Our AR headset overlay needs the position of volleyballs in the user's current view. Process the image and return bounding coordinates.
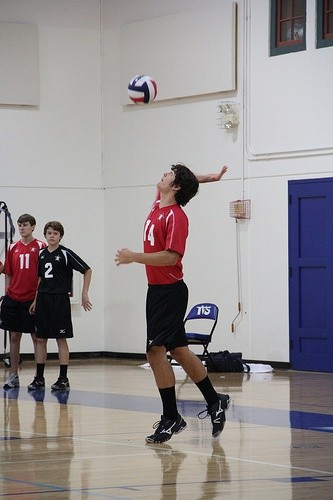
[128,74,158,105]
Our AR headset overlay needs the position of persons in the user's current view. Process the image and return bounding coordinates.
[0,261,4,274]
[27,221,92,390]
[114,163,231,442]
[0,214,47,390]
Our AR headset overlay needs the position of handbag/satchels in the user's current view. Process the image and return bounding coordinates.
[208,350,250,373]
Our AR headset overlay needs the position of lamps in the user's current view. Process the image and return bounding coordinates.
[218,102,240,128]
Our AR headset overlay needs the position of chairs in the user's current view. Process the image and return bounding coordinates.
[169,303,219,371]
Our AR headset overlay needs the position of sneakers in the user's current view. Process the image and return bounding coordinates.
[198,393,230,438]
[3,373,19,387]
[145,413,187,443]
[28,376,45,390]
[51,376,69,390]
